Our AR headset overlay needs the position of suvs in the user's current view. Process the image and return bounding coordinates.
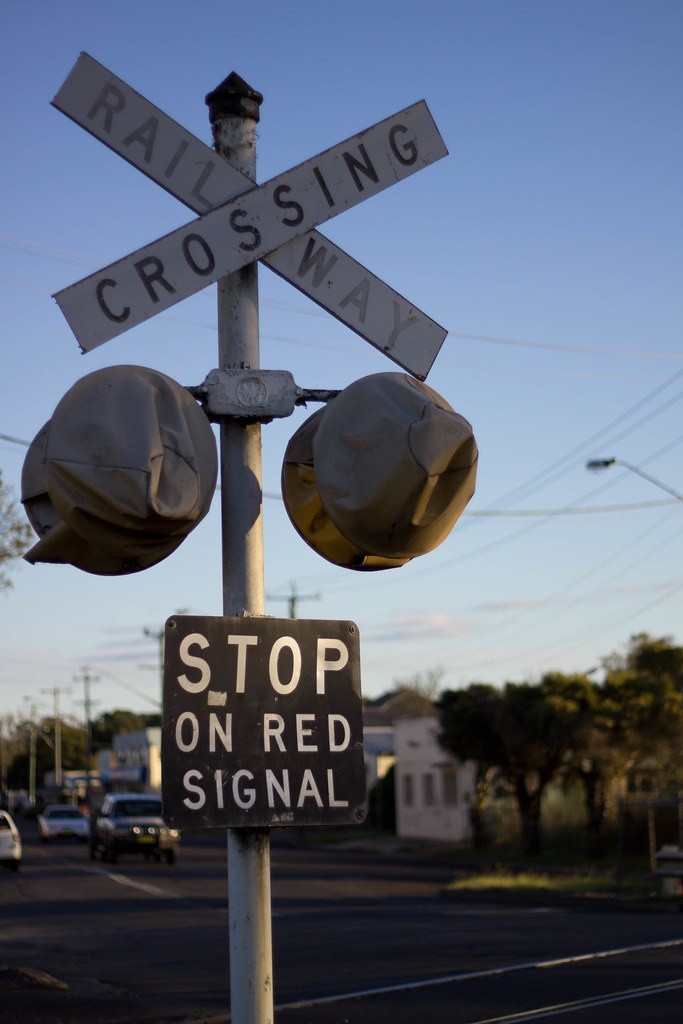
[89,791,181,866]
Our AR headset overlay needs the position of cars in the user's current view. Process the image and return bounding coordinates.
[0,810,22,872]
[37,804,89,844]
